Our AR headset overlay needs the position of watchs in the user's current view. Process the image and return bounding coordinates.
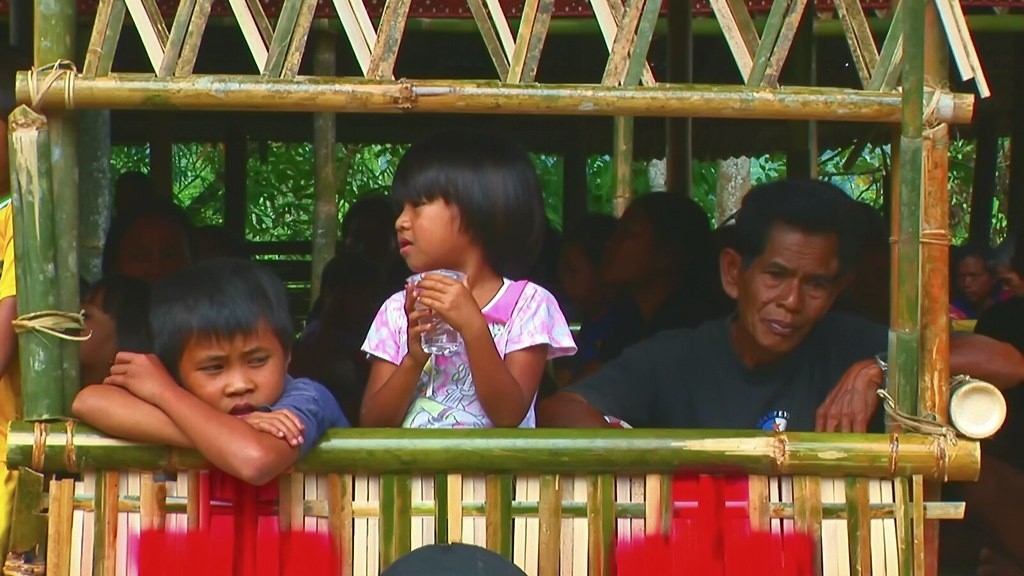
[872,350,888,392]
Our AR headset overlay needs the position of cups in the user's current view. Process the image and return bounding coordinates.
[406,269,469,355]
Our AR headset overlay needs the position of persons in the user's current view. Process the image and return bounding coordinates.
[288,130,1023,576]
[70,252,352,486]
[0,107,228,576]
